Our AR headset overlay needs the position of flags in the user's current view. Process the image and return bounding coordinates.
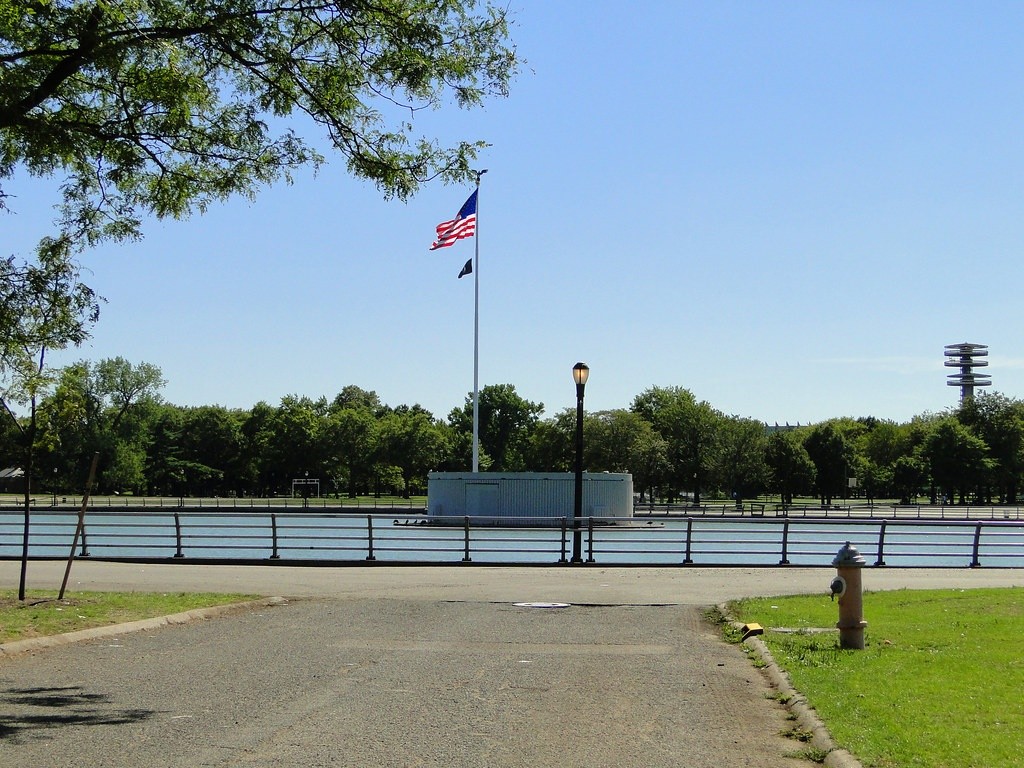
[458,258,473,278]
[458,258,472,278]
[429,187,478,251]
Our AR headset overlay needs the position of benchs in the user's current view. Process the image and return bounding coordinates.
[757,496,778,503]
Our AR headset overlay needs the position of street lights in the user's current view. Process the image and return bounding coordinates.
[571,360,590,564]
[53,468,58,505]
[180,469,184,506]
[305,471,309,508]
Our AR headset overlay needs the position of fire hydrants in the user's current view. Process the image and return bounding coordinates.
[824,542,867,649]
